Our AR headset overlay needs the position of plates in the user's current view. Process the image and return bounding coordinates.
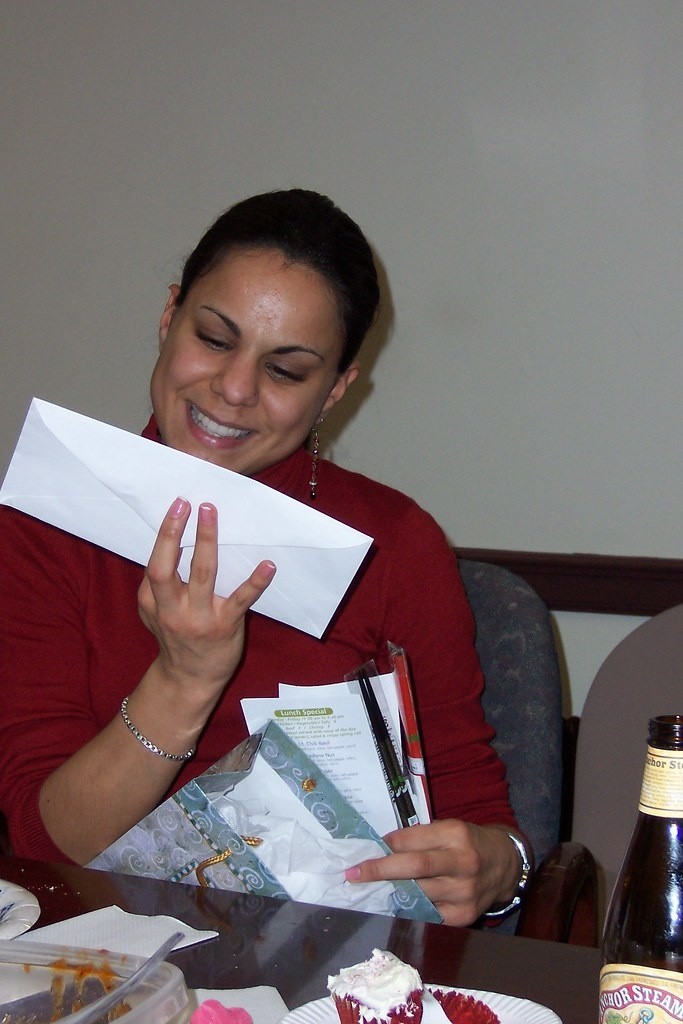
[278,983,562,1023]
[0,879,41,941]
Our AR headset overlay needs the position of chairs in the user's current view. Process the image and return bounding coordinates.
[448,560,683,951]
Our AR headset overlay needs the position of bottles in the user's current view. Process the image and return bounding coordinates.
[598,715,683,1024]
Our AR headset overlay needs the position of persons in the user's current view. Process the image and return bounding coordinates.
[0,185,532,931]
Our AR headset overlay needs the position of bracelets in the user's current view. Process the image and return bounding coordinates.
[482,827,532,919]
[118,695,198,763]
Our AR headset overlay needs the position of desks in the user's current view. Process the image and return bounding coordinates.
[0,854,683,1024]
